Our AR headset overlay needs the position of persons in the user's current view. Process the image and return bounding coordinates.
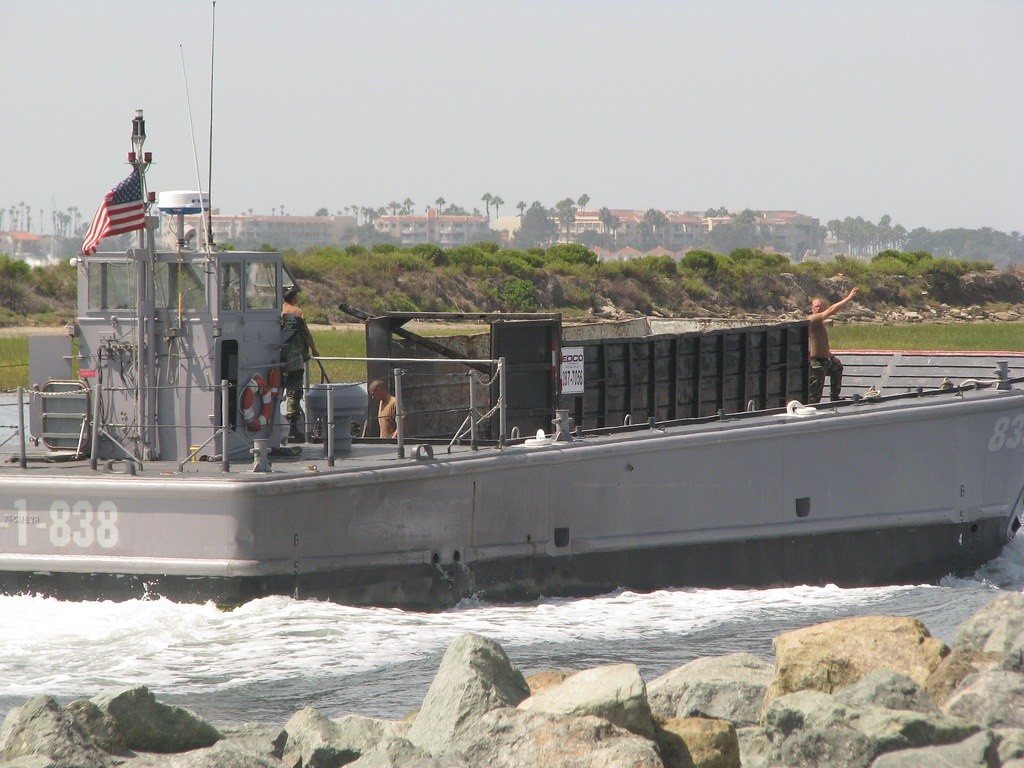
[809,287,861,403]
[370,380,407,438]
[283,288,319,437]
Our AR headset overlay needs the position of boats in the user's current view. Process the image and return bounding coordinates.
[0,0,1023,612]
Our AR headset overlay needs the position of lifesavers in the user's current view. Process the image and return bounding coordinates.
[243,377,272,430]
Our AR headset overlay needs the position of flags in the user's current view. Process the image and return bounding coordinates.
[82,170,145,256]
